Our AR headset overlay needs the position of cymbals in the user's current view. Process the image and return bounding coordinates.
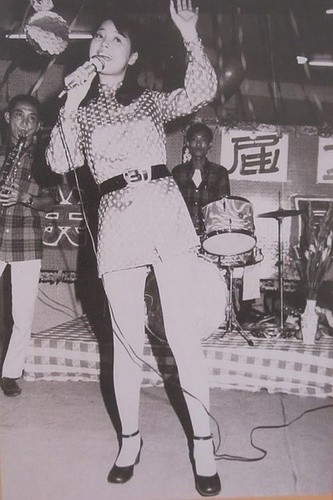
[256,209,302,218]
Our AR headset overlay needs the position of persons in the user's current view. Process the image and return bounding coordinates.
[0,94,58,398]
[170,122,264,323]
[46,0,221,496]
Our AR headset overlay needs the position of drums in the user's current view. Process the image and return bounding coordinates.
[200,196,256,256]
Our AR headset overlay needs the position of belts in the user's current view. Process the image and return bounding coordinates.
[97,163,173,195]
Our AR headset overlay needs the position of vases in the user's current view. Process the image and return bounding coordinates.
[301,300,318,344]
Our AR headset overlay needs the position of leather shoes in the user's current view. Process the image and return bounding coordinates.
[192,434,221,497]
[0,377,22,397]
[107,430,144,484]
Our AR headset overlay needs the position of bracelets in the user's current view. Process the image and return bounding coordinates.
[26,194,33,206]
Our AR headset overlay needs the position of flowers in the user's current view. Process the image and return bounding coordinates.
[293,203,333,300]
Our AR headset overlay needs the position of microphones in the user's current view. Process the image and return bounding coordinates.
[58,55,105,98]
